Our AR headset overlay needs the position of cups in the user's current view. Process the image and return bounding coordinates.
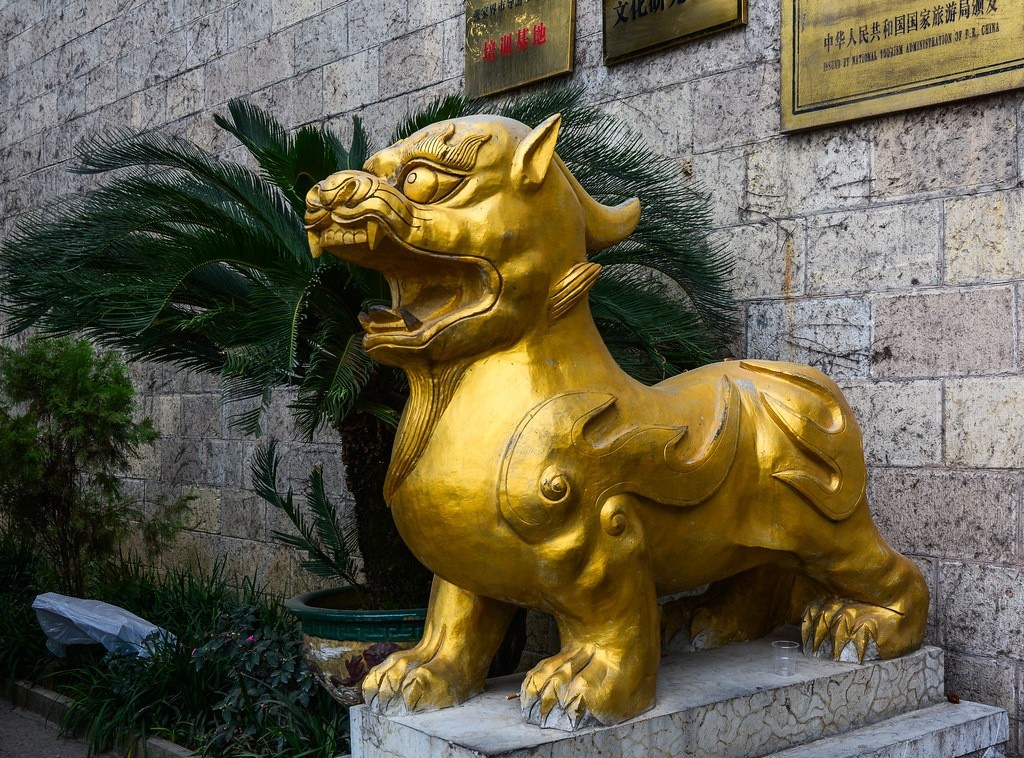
[770,640,800,678]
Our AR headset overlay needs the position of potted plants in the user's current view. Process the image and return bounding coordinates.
[0,74,738,716]
